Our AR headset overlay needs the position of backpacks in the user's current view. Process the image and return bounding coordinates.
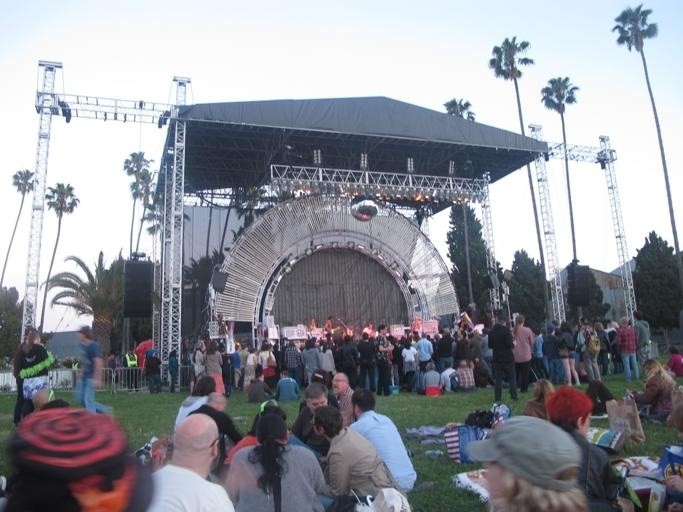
[588,335,601,353]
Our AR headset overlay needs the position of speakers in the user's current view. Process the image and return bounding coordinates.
[123,261,153,318]
[567,265,590,306]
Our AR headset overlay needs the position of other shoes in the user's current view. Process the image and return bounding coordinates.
[477,379,529,402]
[357,381,425,398]
[547,369,638,388]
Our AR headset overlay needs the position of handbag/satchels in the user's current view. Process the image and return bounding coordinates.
[424,373,443,397]
[225,384,232,398]
[449,377,459,392]
[595,397,646,452]
[440,403,511,464]
[616,443,683,504]
[585,379,614,416]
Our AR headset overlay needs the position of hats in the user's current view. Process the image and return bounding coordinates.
[467,414,582,491]
[547,323,555,333]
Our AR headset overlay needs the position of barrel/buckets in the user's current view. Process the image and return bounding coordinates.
[660,444,682,500]
[389,385,401,394]
[424,386,442,396]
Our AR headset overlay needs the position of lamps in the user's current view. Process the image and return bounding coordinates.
[268,175,486,206]
